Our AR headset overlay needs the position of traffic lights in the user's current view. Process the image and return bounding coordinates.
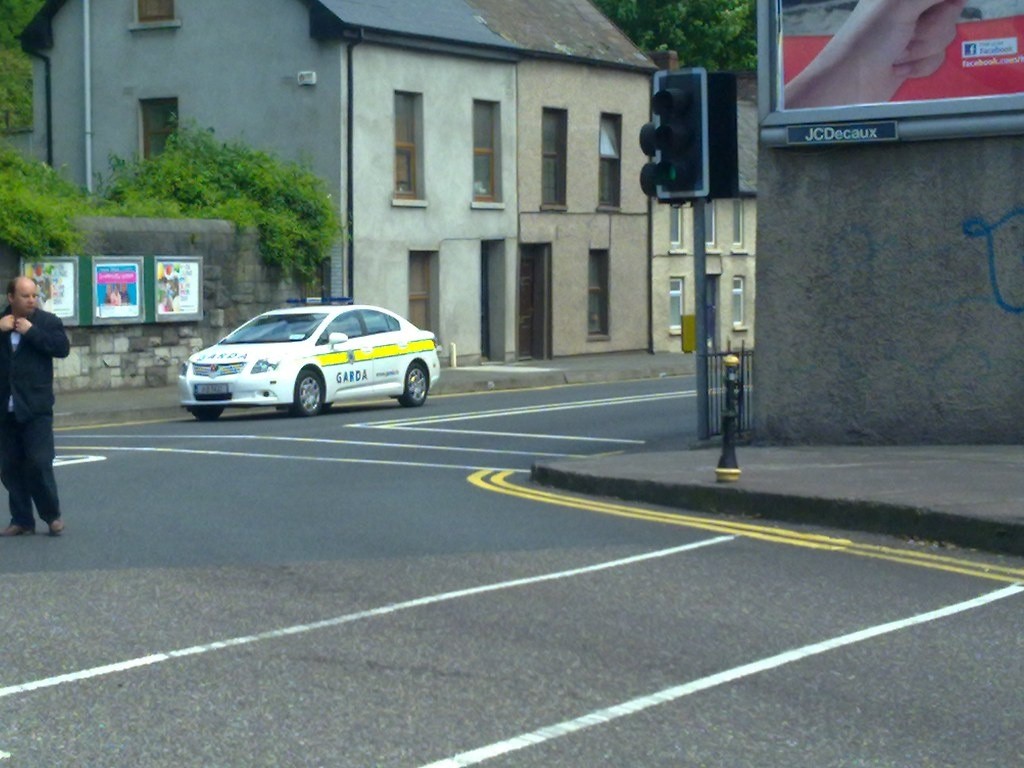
[640,121,657,199]
[652,69,709,199]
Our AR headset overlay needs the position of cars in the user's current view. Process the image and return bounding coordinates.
[175,296,445,415]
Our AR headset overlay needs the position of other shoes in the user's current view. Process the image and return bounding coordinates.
[0,524,35,536]
[49,520,64,534]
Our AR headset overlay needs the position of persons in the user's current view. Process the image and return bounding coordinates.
[785,0,966,109]
[0,276,69,537]
[105,282,130,306]
